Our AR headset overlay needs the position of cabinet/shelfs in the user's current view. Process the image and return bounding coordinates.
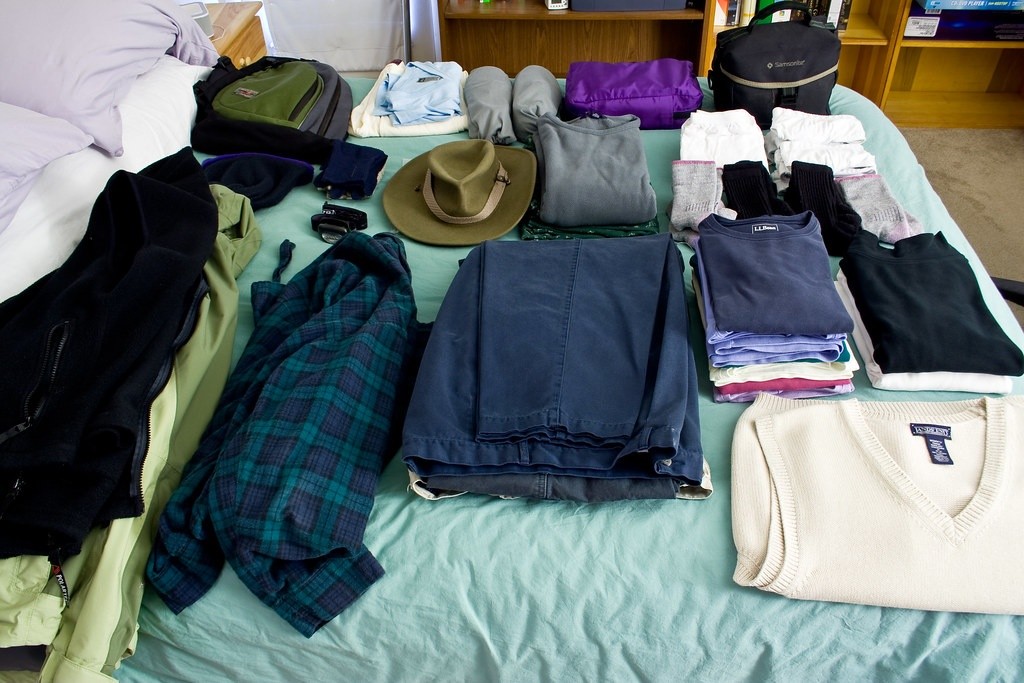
[438,0,711,77]
[703,0,906,109]
[204,1,267,70]
[880,0,1024,129]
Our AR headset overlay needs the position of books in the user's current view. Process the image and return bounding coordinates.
[714,0,852,33]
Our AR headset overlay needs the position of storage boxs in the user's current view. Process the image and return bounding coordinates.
[571,0,686,13]
[904,3,1024,42]
[917,0,1024,10]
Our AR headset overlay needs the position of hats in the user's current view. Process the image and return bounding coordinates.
[200,151,314,212]
[383,140,536,246]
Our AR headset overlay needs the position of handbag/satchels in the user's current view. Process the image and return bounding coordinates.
[565,58,704,130]
[192,56,353,141]
[707,2,841,129]
